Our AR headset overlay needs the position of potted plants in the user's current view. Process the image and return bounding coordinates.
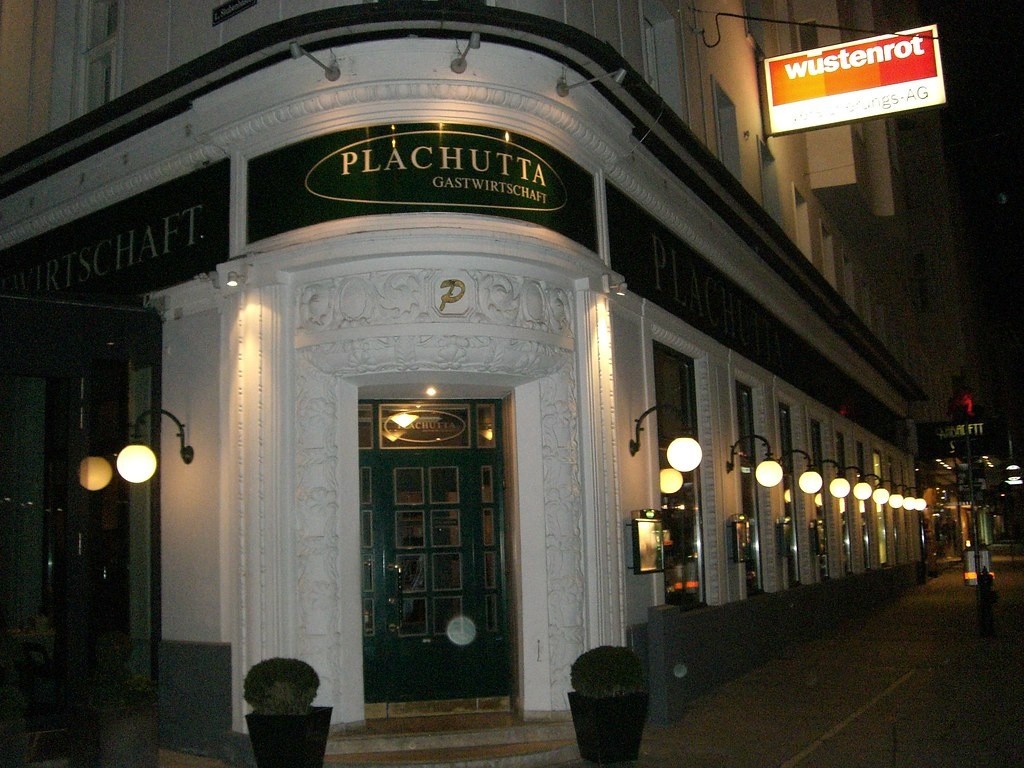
[243,656,333,768]
[567,645,651,765]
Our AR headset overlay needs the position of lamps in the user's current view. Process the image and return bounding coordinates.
[116,407,194,484]
[727,433,784,487]
[843,466,872,501]
[556,68,627,97]
[895,483,916,511]
[876,480,904,508]
[450,31,481,72]
[903,487,927,511]
[865,472,890,505]
[289,40,341,82]
[817,459,849,499]
[628,403,703,473]
[777,448,823,495]
